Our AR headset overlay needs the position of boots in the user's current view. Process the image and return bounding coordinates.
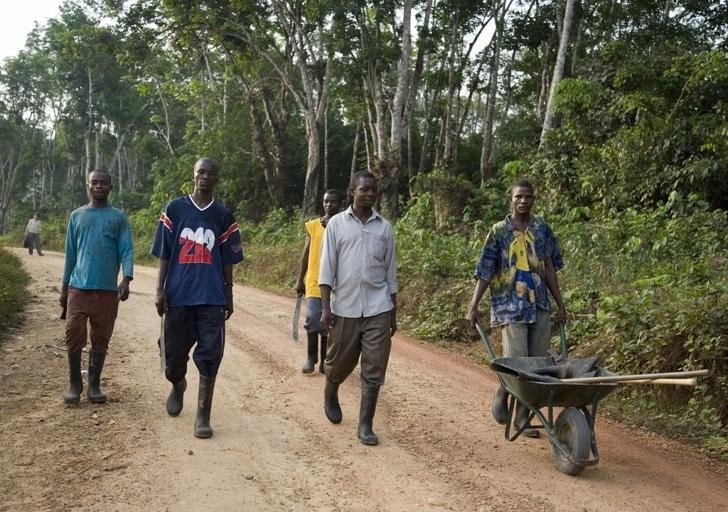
[319,336,327,374]
[492,384,509,425]
[194,376,215,438]
[166,378,186,417]
[514,399,540,438]
[302,331,318,374]
[324,376,342,424]
[87,350,107,404]
[358,386,380,445]
[64,351,83,403]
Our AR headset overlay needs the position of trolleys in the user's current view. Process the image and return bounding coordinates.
[474,320,619,476]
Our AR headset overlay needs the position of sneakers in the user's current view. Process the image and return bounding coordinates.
[29,252,33,255]
[39,253,44,256]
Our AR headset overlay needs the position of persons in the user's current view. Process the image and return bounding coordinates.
[318,171,399,445]
[25,213,44,256]
[295,188,342,373]
[464,179,566,438]
[59,169,134,404]
[149,157,243,438]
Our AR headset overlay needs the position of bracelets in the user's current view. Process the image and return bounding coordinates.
[224,281,233,288]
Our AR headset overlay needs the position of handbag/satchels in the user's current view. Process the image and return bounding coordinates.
[24,235,29,248]
